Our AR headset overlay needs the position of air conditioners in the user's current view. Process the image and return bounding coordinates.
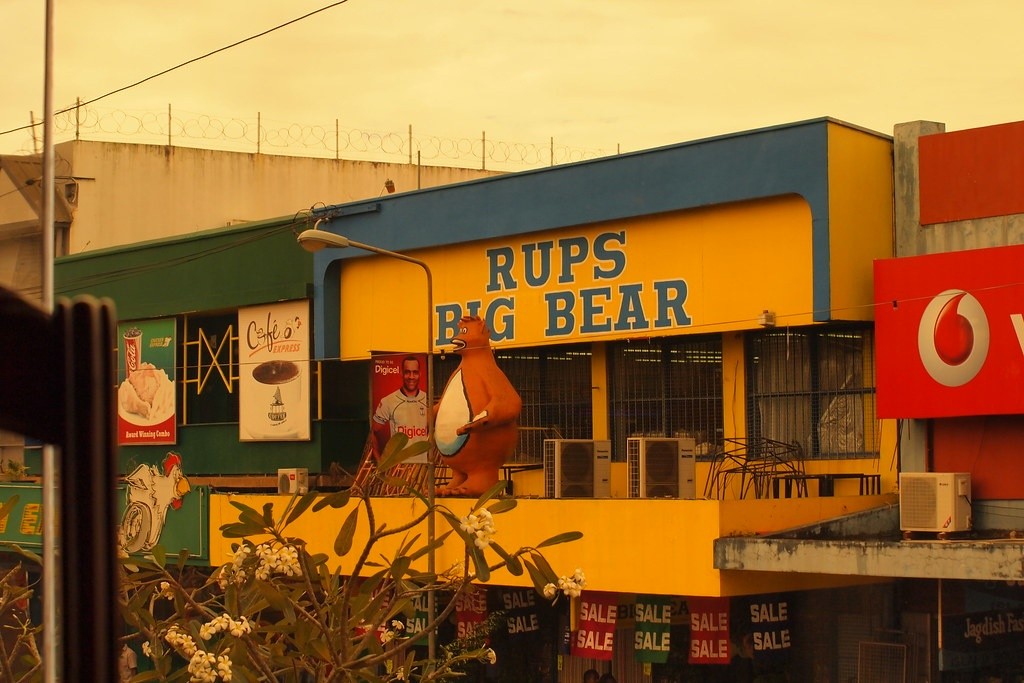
[899,472,972,532]
[627,437,696,500]
[277,468,308,494]
[543,439,611,499]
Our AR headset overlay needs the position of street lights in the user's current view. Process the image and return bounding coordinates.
[296,229,435,660]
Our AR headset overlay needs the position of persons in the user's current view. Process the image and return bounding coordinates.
[368,357,430,465]
